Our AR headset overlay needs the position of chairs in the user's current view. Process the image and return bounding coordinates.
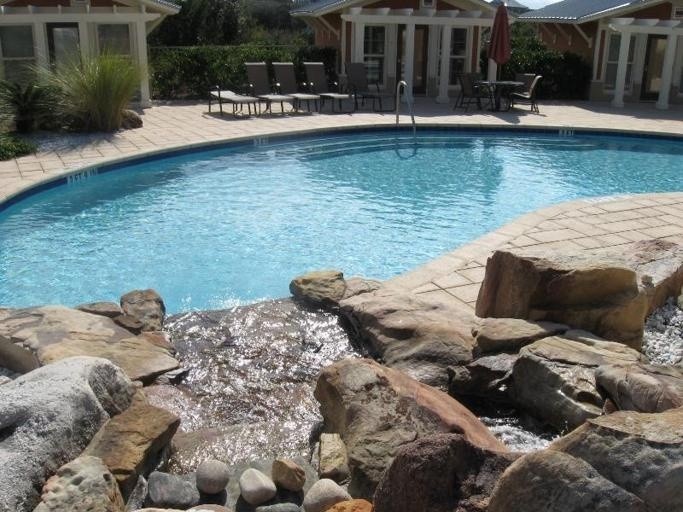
[454,72,542,114]
[209,61,401,119]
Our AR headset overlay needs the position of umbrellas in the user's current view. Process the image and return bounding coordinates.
[486,1,510,111]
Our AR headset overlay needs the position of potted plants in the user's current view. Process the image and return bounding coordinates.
[0,80,61,133]
